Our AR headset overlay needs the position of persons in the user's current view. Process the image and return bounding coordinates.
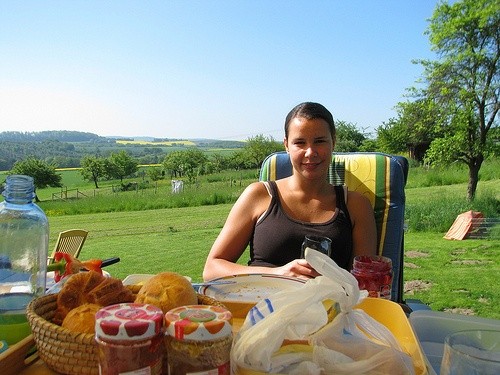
[203,102,377,283]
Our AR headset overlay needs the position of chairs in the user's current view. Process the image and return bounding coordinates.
[47,229,88,264]
[260,152,432,317]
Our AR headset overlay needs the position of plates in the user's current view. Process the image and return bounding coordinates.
[46,269,111,290]
[198,273,310,302]
[122,273,193,287]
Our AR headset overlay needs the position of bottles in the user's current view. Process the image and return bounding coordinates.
[0,175,50,356]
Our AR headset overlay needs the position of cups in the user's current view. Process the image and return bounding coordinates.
[439,330,500,375]
[299,234,332,259]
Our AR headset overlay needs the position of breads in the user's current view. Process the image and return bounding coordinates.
[53,271,198,333]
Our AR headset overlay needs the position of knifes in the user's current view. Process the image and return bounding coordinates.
[191,280,237,286]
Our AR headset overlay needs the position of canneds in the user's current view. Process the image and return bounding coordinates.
[95,304,166,375]
[164,305,234,375]
[351,255,393,301]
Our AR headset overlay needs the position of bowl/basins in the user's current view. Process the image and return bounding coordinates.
[237,297,499,375]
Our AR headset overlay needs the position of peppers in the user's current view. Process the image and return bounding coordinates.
[46,253,103,283]
